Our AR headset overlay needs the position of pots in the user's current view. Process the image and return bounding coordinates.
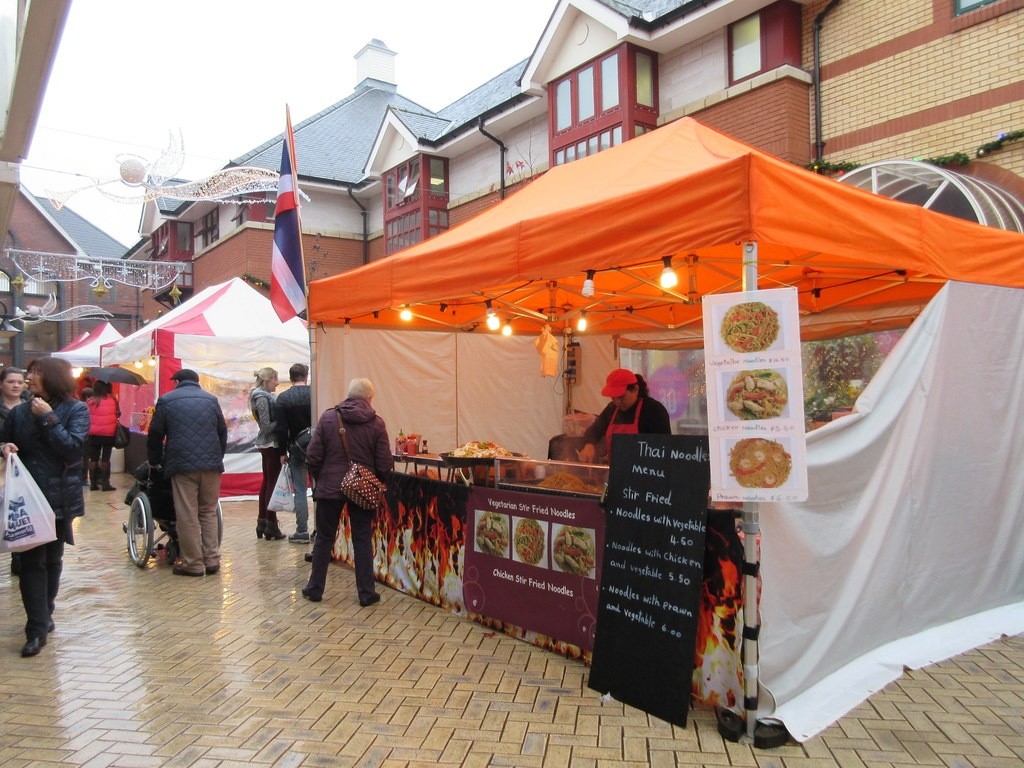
[438,451,523,465]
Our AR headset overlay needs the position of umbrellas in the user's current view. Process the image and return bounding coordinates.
[90,367,147,385]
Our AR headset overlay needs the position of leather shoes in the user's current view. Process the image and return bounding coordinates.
[48,615,55,632]
[22,636,46,656]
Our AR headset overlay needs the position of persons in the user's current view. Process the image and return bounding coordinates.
[578,369,672,463]
[147,369,228,575]
[123,438,166,532]
[0,358,90,655]
[0,367,28,433]
[249,368,286,540]
[302,377,393,607]
[79,377,120,491]
[276,363,310,543]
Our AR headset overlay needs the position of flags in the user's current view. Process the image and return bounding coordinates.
[271,129,307,323]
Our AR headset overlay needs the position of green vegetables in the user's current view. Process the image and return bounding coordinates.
[572,530,584,537]
[492,516,502,522]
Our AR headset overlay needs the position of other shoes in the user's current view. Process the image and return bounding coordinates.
[206,565,220,574]
[360,592,380,605]
[173,561,203,576]
[302,586,322,602]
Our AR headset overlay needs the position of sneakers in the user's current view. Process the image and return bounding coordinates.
[289,531,309,544]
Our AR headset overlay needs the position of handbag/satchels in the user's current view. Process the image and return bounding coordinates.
[0,452,58,553]
[114,424,130,449]
[266,462,296,512]
[341,462,388,511]
[288,427,311,463]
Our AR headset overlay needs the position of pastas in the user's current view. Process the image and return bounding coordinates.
[721,301,779,352]
[538,472,586,492]
[516,518,543,564]
[727,369,787,419]
[728,438,791,488]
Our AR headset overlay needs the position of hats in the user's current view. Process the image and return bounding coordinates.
[601,368,638,396]
[170,369,199,383]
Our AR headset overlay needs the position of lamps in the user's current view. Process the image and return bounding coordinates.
[0,301,23,337]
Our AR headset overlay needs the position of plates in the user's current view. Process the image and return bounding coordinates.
[551,522,596,580]
[473,509,509,558]
[718,432,797,491]
[707,295,786,354]
[714,363,792,423]
[511,515,548,569]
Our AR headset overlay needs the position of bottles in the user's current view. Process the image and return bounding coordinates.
[395,428,428,456]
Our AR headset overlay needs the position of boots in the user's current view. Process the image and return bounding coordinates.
[264,520,286,540]
[256,518,266,538]
[101,462,116,491]
[88,462,100,490]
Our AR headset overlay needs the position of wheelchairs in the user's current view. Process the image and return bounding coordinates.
[123,461,222,568]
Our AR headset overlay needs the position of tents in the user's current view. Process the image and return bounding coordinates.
[99,278,311,502]
[306,115,1024,743]
[50,321,124,368]
